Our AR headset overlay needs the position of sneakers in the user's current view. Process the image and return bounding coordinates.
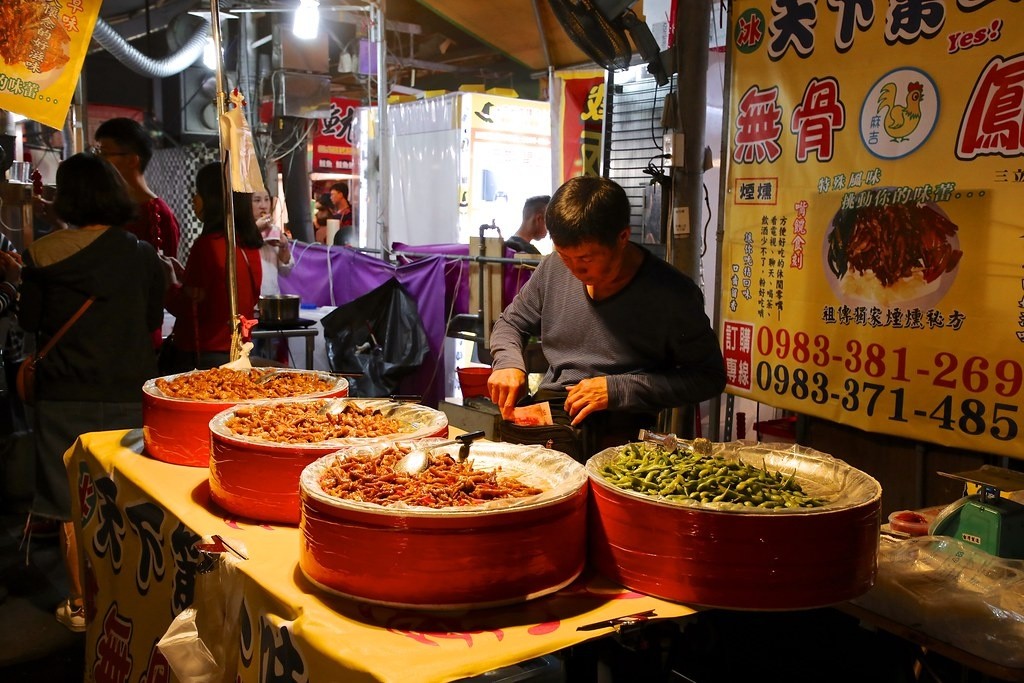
[55,599,86,632]
[23,517,60,537]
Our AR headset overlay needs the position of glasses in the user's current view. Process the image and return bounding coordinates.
[95,148,130,159]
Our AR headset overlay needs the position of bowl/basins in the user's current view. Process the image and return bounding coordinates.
[888,511,935,536]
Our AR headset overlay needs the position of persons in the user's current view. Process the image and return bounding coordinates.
[157,162,264,377]
[34,117,180,361]
[251,185,294,364]
[18,153,162,632]
[504,196,552,310]
[313,182,352,244]
[0,145,24,600]
[487,176,728,464]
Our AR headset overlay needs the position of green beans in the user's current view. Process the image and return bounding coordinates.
[598,440,831,509]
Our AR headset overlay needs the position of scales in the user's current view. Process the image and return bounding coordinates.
[928,465,1024,567]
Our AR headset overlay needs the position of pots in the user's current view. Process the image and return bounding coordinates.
[259,294,300,321]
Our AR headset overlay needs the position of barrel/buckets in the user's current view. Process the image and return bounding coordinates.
[454,366,493,398]
[288,303,338,372]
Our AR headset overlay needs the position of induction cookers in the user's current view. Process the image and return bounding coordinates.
[253,318,317,329]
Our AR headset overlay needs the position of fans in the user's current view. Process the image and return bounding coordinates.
[548,0,680,87]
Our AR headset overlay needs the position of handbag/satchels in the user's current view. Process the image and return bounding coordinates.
[17,354,35,401]
[497,393,595,465]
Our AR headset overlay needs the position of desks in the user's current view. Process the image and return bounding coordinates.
[61,426,706,683]
[250,326,319,370]
[836,504,1024,683]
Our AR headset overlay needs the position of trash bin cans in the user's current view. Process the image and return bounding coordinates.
[444,313,480,397]
